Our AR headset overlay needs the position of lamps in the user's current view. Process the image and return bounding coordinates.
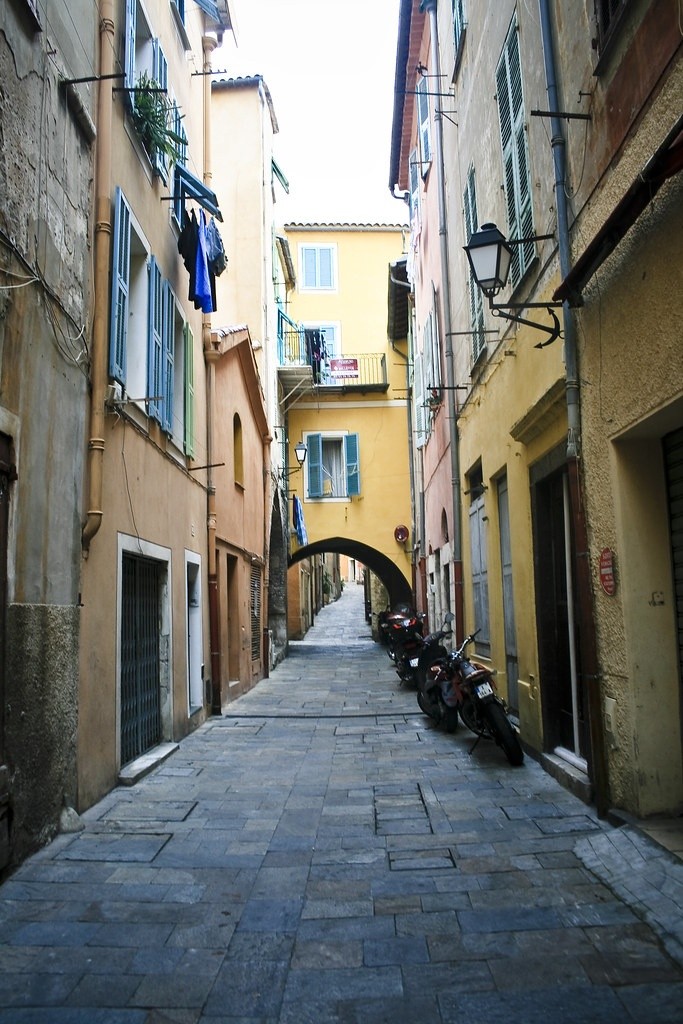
[279,441,309,478]
[462,223,571,311]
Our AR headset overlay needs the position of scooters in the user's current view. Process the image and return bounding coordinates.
[415,612,458,734]
[372,605,426,690]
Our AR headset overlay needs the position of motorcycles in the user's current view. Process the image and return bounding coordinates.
[433,628,524,767]
